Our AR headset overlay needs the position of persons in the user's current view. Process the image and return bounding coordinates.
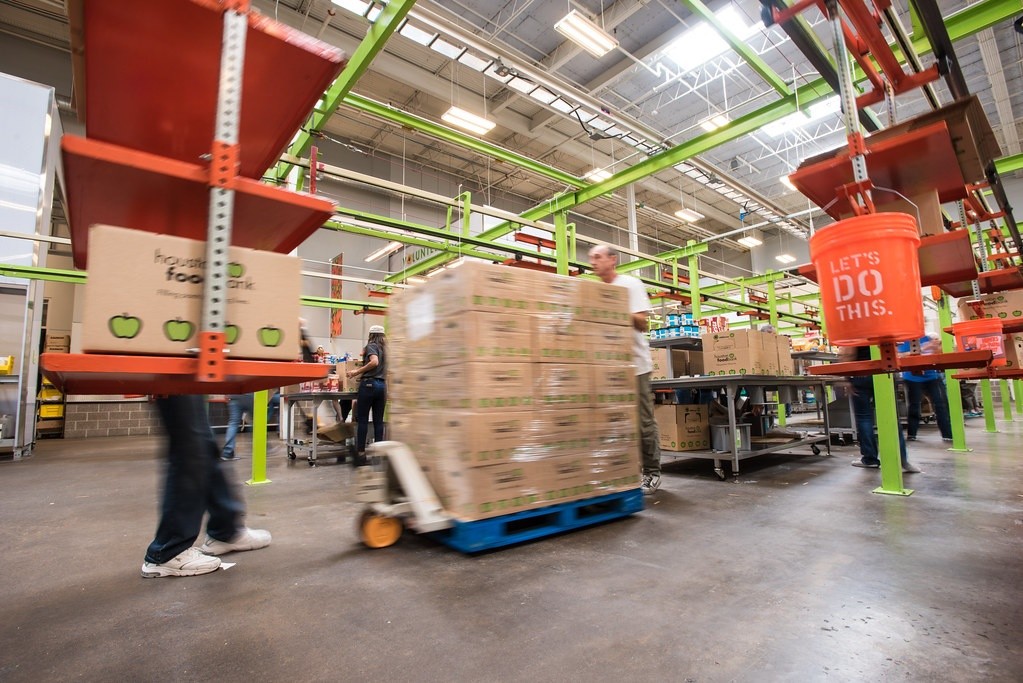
[838,346,921,473]
[221,392,253,461]
[958,379,983,418]
[897,335,953,441]
[587,244,662,495]
[345,325,387,468]
[139,392,272,578]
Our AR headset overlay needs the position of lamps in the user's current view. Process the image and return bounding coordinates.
[775,228,797,263]
[674,173,705,223]
[553,0,619,61]
[441,60,497,136]
[737,216,763,248]
[584,136,615,183]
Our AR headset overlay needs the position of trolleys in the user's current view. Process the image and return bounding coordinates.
[357,438,647,555]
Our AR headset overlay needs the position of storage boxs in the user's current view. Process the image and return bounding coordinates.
[957,288,1023,321]
[654,403,711,452]
[383,257,643,523]
[0,355,15,375]
[701,328,780,376]
[336,362,363,380]
[37,334,71,420]
[648,347,686,380]
[309,422,356,443]
[710,422,751,452]
[80,222,303,363]
[339,377,361,392]
[284,376,339,395]
[649,313,730,341]
[774,335,796,376]
[956,332,1023,374]
[839,191,944,238]
[671,349,703,377]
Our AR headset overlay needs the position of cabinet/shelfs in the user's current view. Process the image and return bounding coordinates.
[789,119,995,377]
[937,265,1023,381]
[39,0,349,396]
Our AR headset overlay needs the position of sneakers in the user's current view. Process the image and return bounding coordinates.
[141,546,221,578]
[642,474,661,494]
[202,525,272,554]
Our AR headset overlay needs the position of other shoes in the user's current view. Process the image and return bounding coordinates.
[907,435,917,441]
[852,459,878,468]
[963,409,982,417]
[357,451,366,460]
[220,452,234,460]
[904,463,921,473]
[943,434,952,439]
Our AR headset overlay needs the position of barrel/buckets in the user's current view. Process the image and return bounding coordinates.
[807,184,926,347]
[953,301,1006,367]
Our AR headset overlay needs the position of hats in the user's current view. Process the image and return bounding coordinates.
[369,325,384,334]
